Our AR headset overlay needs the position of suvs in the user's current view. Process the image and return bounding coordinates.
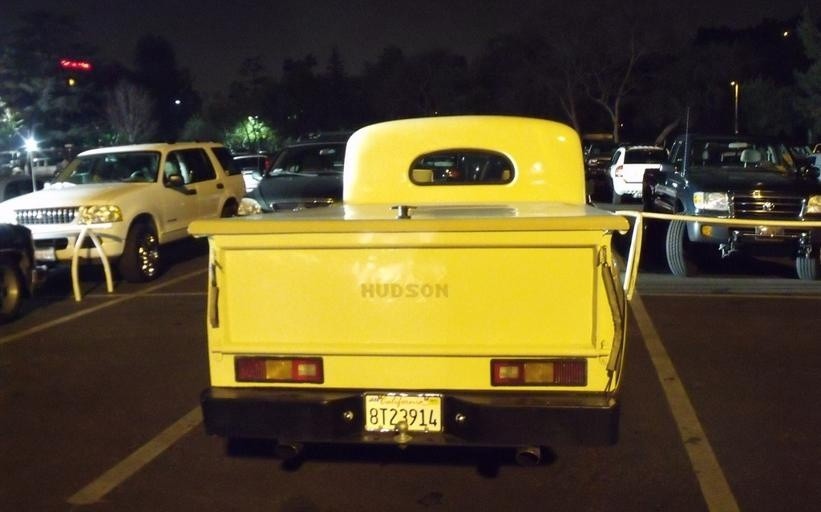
[0,140,246,283]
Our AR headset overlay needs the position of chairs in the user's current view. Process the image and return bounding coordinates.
[410,169,434,184]
[739,148,762,166]
[152,161,178,179]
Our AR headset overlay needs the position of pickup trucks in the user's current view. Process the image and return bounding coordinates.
[186,111,630,468]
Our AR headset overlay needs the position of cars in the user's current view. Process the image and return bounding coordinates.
[233,153,268,194]
[575,126,821,286]
[1,220,35,324]
[0,132,90,201]
[237,140,347,219]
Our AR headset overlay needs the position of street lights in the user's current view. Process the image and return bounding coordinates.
[729,77,738,134]
